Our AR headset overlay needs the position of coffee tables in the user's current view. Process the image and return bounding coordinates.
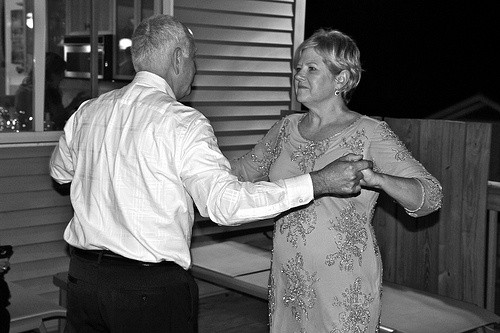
[52,271,68,333]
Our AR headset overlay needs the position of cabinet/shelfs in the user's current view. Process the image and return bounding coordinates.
[64,0,112,36]
[113,0,163,80]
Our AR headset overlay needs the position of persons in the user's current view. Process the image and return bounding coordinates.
[229,28,444,333]
[49,14,373,333]
[15,52,88,130]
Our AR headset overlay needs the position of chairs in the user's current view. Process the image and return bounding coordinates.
[4,281,67,333]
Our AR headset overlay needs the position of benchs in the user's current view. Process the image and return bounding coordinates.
[190,239,500,333]
